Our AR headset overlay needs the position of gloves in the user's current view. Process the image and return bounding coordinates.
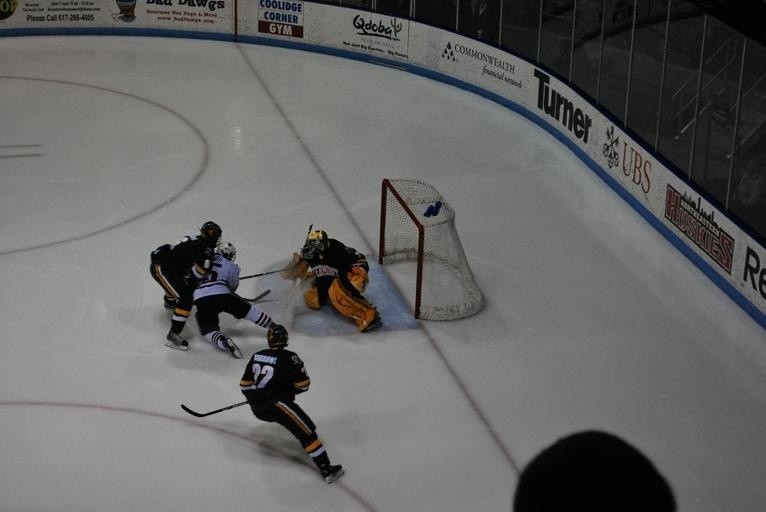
[280,254,309,280]
[348,267,370,293]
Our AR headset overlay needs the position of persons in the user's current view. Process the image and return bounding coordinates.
[238,324,342,478]
[192,239,274,357]
[297,229,384,332]
[149,220,223,347]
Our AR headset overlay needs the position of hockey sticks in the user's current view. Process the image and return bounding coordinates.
[181,400,249,417]
[238,225,313,280]
[242,290,272,302]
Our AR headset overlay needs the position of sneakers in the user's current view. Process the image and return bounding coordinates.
[167,332,189,346]
[321,464,343,479]
[222,338,241,359]
[359,307,382,332]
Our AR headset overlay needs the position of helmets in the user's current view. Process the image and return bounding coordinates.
[267,324,288,347]
[217,241,236,261]
[199,221,222,249]
[306,230,328,251]
